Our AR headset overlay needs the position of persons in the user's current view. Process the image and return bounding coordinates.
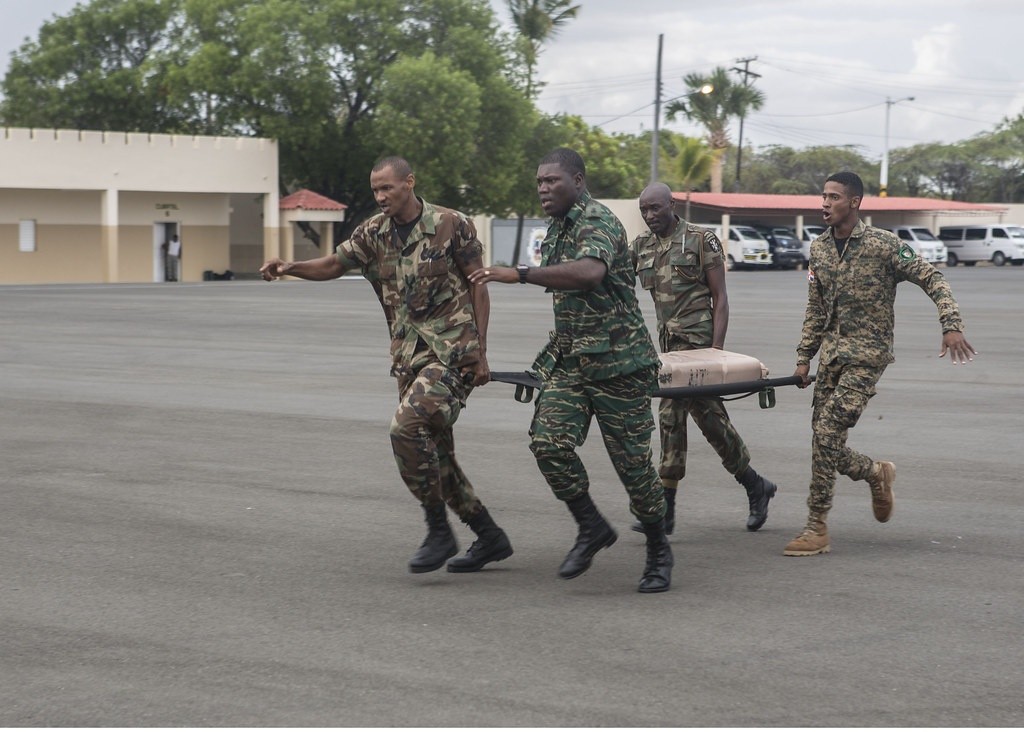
[628,176,779,536]
[771,172,979,557]
[460,148,678,596]
[246,150,518,577]
[162,234,183,281]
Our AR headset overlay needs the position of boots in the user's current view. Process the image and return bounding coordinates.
[783,508,831,556]
[446,503,515,573]
[637,518,675,592]
[406,498,460,574]
[630,479,678,536]
[557,491,619,580]
[736,464,778,532]
[865,460,897,523]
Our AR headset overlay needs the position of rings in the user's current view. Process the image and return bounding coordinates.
[483,270,491,276]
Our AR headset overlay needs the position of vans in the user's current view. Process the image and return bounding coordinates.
[689,224,1023,271]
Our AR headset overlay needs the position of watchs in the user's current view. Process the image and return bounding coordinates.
[515,263,530,285]
